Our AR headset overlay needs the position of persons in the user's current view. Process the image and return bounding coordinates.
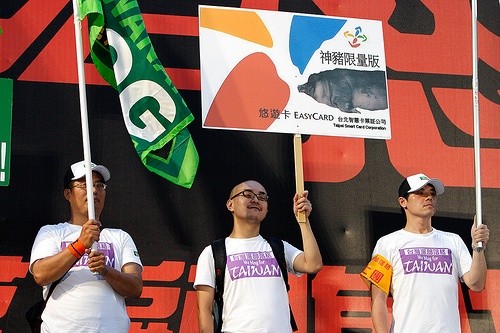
[192,181,323,333]
[29,161,144,332]
[372,172,490,333]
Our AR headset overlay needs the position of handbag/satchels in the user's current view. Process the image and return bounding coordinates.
[26,301,45,333]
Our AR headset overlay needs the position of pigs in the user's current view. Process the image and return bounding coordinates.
[297,68,388,114]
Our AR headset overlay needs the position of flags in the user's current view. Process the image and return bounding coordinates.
[78,0,199,190]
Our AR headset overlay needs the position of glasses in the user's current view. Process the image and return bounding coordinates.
[231,190,268,201]
[70,182,107,190]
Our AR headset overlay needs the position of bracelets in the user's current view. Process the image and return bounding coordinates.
[68,238,86,258]
[472,245,486,251]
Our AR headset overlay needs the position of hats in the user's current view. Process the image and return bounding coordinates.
[399,173,444,198]
[64,160,111,188]
